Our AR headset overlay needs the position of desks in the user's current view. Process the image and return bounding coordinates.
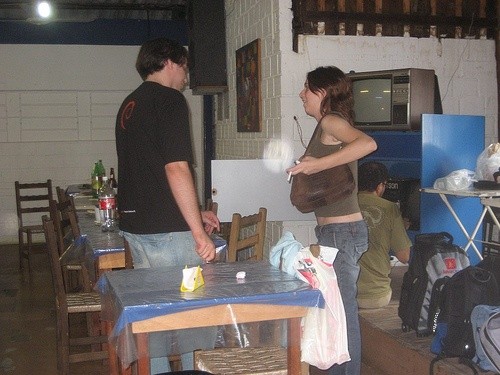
[419,187,500,261]
[92,260,326,375]
[68,185,98,210]
[74,209,228,375]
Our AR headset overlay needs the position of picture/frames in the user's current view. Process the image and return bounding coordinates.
[235,38,263,133]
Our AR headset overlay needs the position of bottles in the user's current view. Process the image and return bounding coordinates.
[99,176,116,232]
[108,167,118,197]
[92,163,101,197]
[98,160,107,185]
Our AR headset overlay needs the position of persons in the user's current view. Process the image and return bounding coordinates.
[116,37,221,375]
[355,161,412,309]
[287,66,377,374]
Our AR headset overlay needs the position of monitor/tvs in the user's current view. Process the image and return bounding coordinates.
[344,68,435,131]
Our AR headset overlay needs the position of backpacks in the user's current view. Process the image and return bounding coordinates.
[429,264,499,358]
[471,305,500,373]
[398,232,470,336]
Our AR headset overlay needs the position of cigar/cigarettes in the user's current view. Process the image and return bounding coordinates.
[287,171,292,182]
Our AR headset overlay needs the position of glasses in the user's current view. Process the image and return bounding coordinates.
[378,181,389,190]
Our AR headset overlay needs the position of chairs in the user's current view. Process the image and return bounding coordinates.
[14,179,268,375]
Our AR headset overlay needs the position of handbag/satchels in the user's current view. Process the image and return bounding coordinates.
[290,113,355,214]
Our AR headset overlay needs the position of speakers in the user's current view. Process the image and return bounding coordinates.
[188,0,227,89]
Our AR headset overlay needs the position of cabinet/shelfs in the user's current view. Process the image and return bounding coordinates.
[358,113,485,266]
